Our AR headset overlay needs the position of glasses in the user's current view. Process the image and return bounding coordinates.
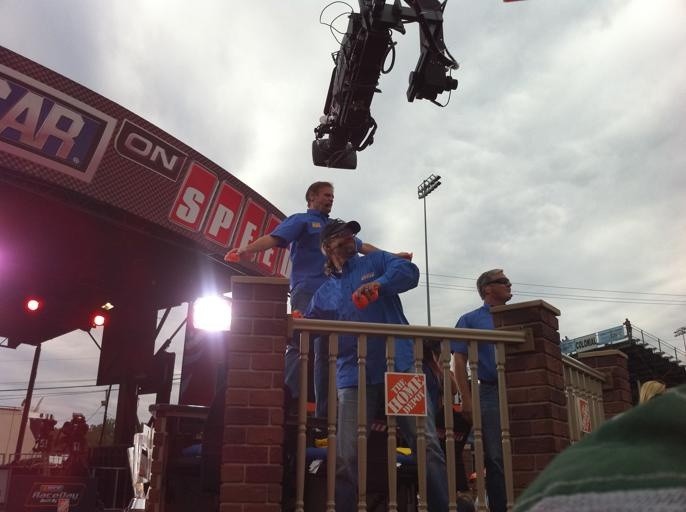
[483,278,509,284]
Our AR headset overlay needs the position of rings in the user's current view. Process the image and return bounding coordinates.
[364,285,370,290]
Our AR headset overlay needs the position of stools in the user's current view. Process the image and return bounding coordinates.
[149,404,210,511]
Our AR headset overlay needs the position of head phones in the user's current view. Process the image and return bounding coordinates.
[320,239,332,257]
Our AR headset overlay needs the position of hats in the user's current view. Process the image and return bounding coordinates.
[320,219,360,241]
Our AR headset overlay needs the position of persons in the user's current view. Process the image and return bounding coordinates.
[285,312,312,467]
[423,268,513,511]
[292,218,449,512]
[208,372,227,487]
[224,181,412,417]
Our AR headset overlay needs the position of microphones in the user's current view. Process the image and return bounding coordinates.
[327,242,343,256]
